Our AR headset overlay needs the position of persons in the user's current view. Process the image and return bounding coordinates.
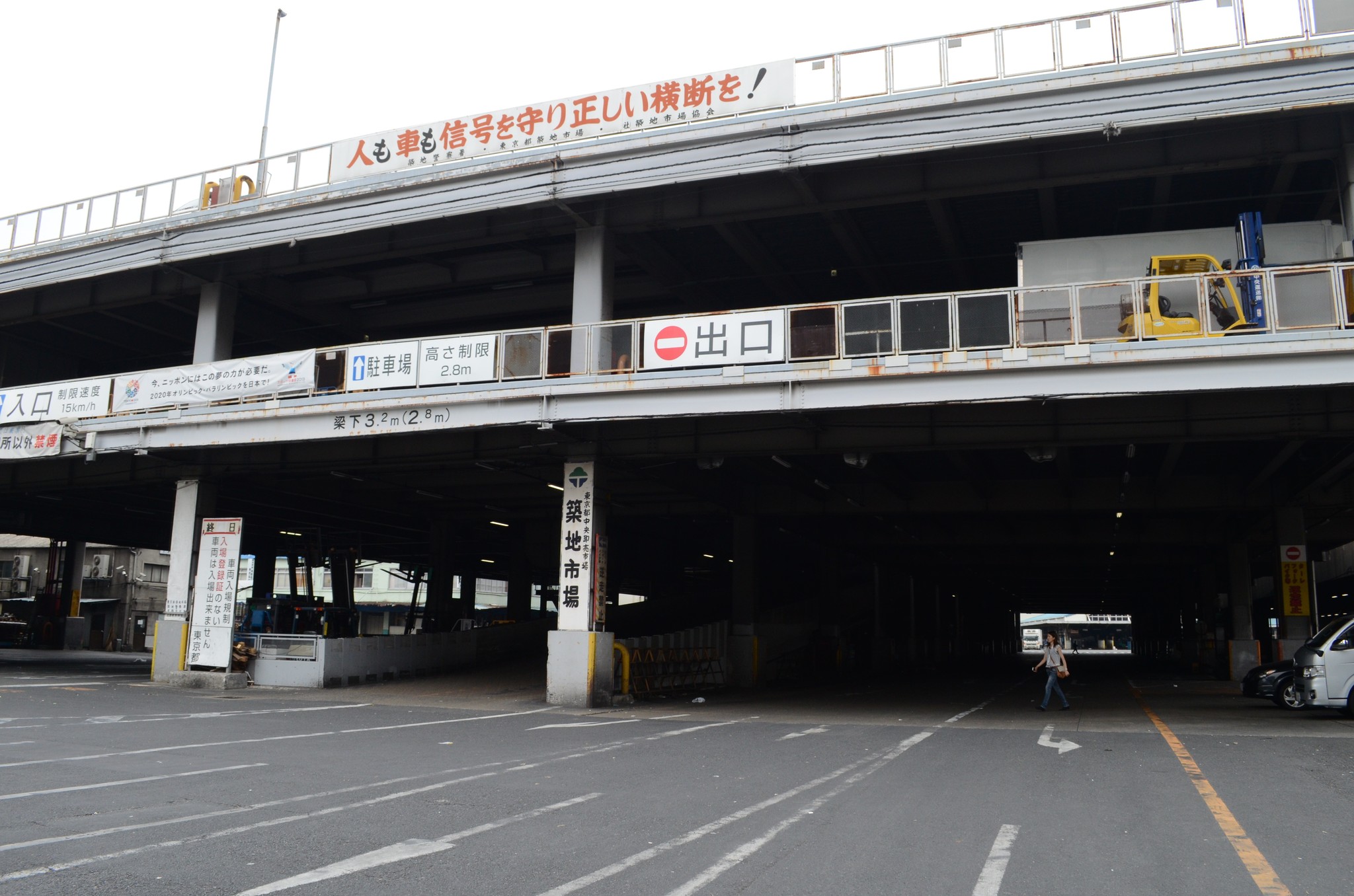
[1073,640,1079,654]
[1034,630,1070,712]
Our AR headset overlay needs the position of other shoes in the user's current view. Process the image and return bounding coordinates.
[1058,706,1070,711]
[1033,701,1044,711]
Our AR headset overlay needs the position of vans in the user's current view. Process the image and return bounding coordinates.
[1293,614,1354,720]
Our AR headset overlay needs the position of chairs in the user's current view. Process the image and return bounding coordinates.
[1158,296,1192,319]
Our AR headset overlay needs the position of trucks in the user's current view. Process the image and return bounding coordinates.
[1023,628,1043,650]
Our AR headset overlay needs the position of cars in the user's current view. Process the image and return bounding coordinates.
[1242,659,1306,710]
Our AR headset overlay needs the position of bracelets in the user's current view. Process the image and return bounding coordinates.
[1065,668,1068,670]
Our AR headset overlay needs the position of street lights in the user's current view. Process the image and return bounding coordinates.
[257,6,288,192]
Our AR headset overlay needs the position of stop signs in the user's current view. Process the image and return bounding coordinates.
[654,325,688,361]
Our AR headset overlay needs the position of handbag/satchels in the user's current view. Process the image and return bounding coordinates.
[1055,665,1070,678]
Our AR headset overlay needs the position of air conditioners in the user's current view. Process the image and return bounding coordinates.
[11,556,30,592]
[91,554,110,578]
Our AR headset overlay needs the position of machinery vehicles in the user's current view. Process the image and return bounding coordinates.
[1115,211,1270,342]
